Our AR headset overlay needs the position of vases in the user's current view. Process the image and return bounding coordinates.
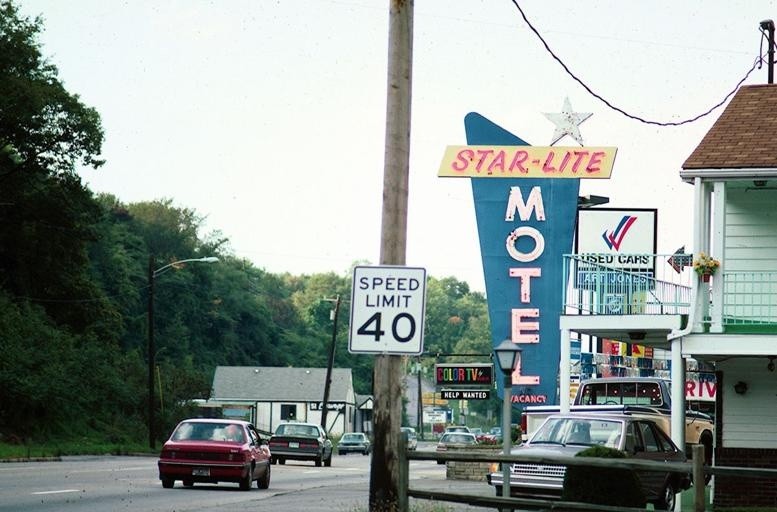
[701,272,711,283]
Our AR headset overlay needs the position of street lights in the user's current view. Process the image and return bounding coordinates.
[493,337,523,512]
[147,257,219,449]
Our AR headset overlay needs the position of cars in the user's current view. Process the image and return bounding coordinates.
[437,426,500,464]
[268,423,332,466]
[158,418,270,489]
[338,433,370,455]
[486,414,690,512]
[401,427,417,450]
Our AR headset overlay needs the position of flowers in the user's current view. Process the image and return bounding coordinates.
[696,253,719,271]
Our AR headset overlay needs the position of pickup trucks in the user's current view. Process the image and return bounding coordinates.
[522,378,713,486]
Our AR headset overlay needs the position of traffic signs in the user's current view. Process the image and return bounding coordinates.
[348,264,427,355]
